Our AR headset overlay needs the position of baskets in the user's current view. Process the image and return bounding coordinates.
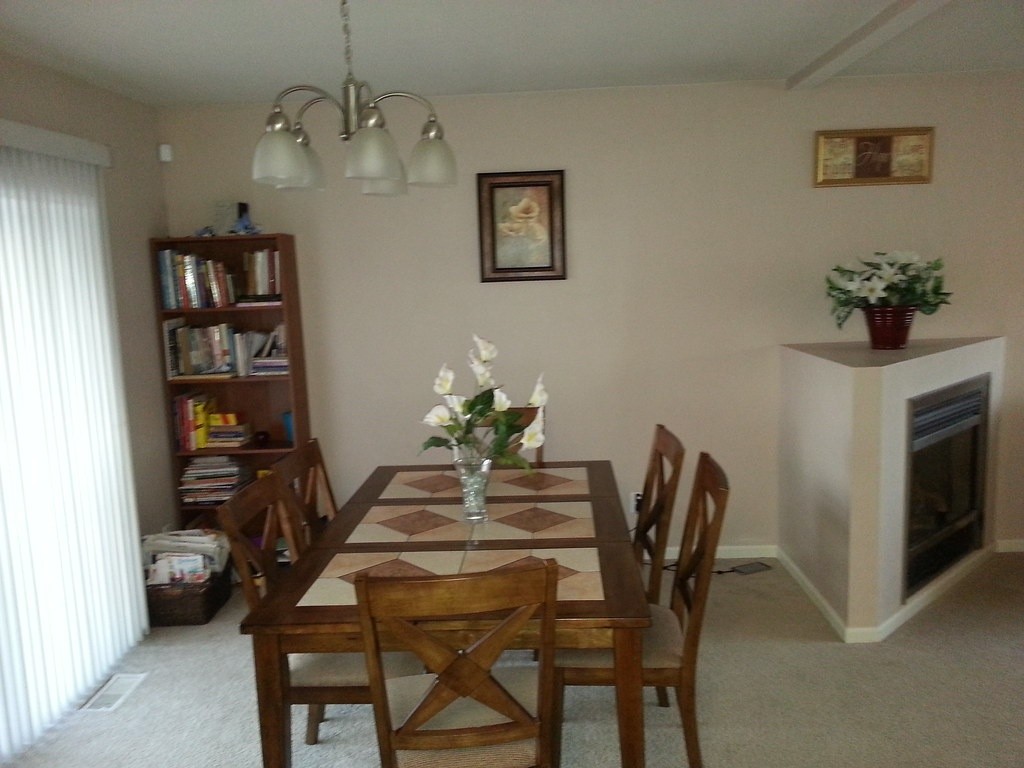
[145,550,234,626]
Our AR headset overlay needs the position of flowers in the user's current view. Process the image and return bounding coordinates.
[416,332,548,476]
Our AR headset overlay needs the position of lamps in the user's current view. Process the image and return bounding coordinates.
[251,0,459,196]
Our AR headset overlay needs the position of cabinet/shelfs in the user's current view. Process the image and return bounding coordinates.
[149,234,310,529]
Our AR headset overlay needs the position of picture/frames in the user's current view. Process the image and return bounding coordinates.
[814,127,936,187]
[477,170,566,283]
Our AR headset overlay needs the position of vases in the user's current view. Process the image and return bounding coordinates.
[455,457,491,521]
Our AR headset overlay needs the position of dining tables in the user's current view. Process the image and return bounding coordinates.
[240,466,653,768]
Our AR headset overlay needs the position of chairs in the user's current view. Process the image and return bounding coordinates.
[271,438,337,546]
[215,472,307,610]
[472,406,545,465]
[556,452,729,768]
[356,559,563,768]
[634,424,685,605]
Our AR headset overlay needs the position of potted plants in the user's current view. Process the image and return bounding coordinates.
[823,252,953,350]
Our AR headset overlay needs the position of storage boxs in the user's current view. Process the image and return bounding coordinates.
[147,566,232,626]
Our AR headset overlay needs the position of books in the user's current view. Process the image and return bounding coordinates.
[157,249,290,504]
